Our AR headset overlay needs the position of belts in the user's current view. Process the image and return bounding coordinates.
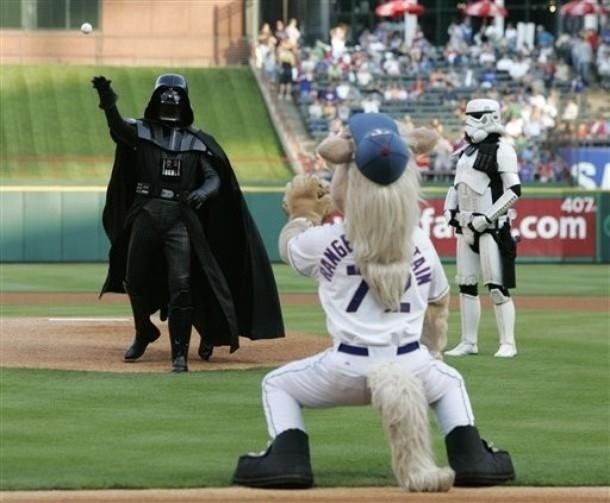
[338,342,418,355]
[136,184,174,199]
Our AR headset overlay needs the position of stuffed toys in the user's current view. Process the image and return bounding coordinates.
[231,109,518,487]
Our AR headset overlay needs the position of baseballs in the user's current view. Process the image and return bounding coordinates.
[80,23,93,36]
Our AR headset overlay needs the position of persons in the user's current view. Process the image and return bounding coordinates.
[443,92,521,357]
[257,16,610,191]
[88,66,222,375]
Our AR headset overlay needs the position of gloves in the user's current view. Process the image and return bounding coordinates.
[187,177,221,209]
[91,76,118,108]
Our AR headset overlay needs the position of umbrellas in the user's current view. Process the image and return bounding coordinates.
[461,0,509,19]
[375,0,429,18]
[557,0,609,17]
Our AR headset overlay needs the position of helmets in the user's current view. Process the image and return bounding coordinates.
[157,87,186,123]
[463,99,506,144]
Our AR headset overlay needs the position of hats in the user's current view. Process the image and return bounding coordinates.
[350,112,409,185]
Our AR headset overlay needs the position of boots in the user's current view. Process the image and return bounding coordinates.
[123,281,160,359]
[168,290,192,372]
[443,292,482,357]
[490,289,517,358]
[233,429,312,484]
[445,424,516,486]
